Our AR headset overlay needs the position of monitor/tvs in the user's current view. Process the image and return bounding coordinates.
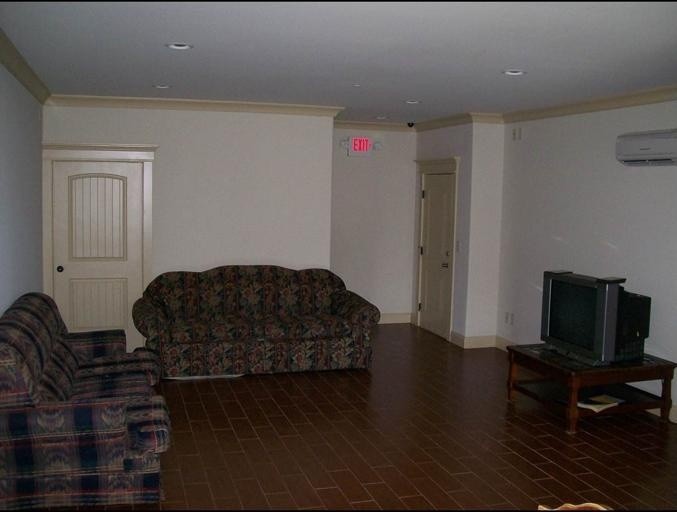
[540,269,653,366]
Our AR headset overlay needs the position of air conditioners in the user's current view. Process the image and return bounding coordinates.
[614,127,677,166]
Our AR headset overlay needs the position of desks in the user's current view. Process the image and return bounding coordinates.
[505,343,677,434]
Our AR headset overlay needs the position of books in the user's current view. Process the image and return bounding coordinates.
[572,393,625,414]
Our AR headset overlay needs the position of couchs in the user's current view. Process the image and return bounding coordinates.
[130,264,380,383]
[1,291,169,511]
